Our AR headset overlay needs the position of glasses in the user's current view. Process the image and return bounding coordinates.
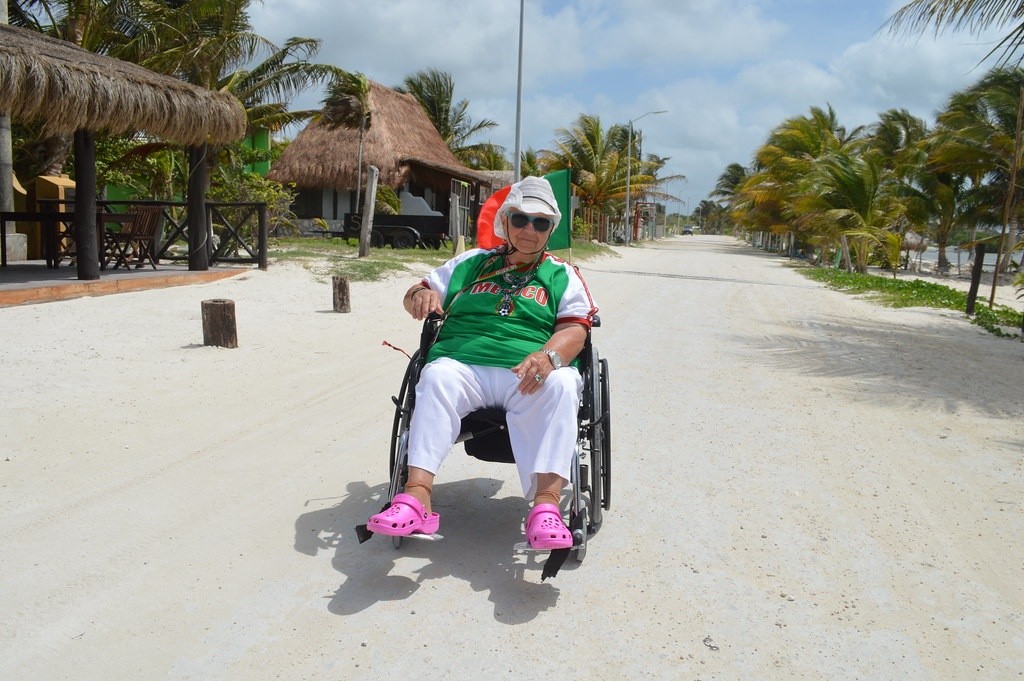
[504,212,555,232]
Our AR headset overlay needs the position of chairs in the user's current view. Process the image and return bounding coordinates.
[105,205,164,268]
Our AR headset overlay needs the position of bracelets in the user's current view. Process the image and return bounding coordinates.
[410,287,426,301]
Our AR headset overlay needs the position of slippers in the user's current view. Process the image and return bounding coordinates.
[525,503,573,550]
[367,493,440,536]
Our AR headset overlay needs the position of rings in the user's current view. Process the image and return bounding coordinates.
[534,374,541,382]
[539,379,544,385]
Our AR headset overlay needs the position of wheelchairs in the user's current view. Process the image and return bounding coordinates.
[355,309,613,564]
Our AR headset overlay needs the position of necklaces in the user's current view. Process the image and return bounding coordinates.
[499,248,548,317]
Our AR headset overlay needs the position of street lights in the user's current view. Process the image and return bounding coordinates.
[625,110,669,247]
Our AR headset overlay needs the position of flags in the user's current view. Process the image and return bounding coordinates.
[477,166,572,252]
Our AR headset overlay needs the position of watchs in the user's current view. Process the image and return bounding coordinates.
[540,348,562,370]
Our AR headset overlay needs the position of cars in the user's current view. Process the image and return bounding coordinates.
[682,225,694,236]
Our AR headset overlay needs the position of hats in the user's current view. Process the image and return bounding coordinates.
[494,176,562,239]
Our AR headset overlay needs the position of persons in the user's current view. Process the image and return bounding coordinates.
[366,175,599,550]
